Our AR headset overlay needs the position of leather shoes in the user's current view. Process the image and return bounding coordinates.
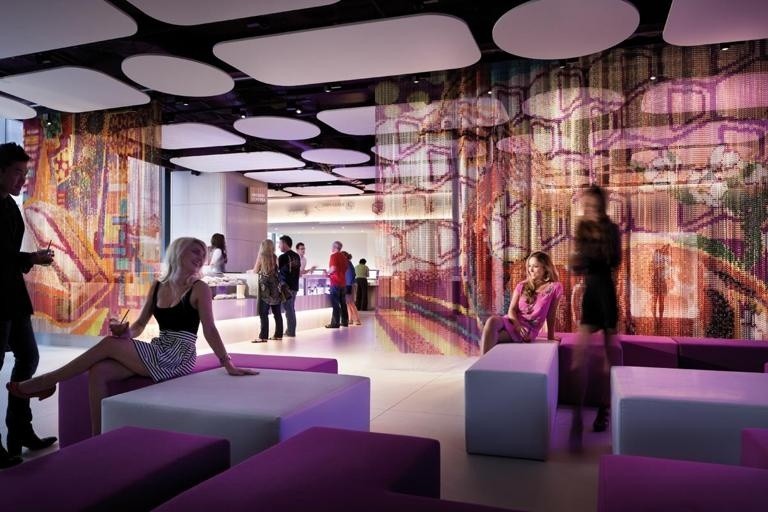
[593,403,610,430]
[0,445,23,467]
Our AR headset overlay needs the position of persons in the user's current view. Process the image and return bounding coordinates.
[294,242,316,276]
[566,184,621,454]
[3,235,258,450]
[252,239,284,343]
[277,234,298,338]
[324,241,369,328]
[649,244,666,329]
[480,252,561,359]
[0,141,58,465]
[210,232,228,272]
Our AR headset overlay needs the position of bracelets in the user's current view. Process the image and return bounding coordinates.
[218,354,231,362]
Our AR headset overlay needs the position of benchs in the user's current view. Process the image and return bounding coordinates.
[672,336,766,373]
[1,423,234,511]
[353,490,505,511]
[169,352,339,375]
[466,343,559,462]
[599,453,766,512]
[612,334,677,367]
[611,364,766,465]
[525,331,622,407]
[58,359,158,449]
[739,427,767,470]
[100,365,370,466]
[151,424,441,510]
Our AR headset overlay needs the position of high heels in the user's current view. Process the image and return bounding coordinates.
[8,428,57,455]
[6,380,55,401]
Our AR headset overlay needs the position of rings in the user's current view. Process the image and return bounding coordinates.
[41,258,50,268]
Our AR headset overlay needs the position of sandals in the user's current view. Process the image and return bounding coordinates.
[252,339,266,343]
[269,336,281,340]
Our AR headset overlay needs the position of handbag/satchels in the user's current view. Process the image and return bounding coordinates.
[277,281,293,303]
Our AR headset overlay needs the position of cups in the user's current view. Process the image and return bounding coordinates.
[523,332,533,342]
[236,285,246,299]
[109,319,129,337]
[37,248,53,267]
